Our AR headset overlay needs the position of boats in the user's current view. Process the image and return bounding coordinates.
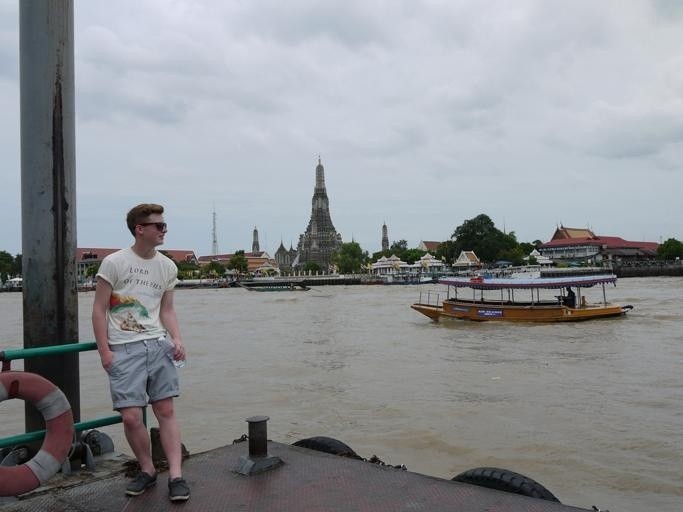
[0,274,23,291]
[410,275,633,322]
[238,283,311,292]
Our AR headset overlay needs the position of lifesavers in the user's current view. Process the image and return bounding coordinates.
[1,370,73,496]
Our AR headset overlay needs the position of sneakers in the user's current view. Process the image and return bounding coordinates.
[169,475,192,503]
[125,469,159,495]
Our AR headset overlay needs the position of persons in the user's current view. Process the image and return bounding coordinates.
[89,203,190,501]
[566,287,575,307]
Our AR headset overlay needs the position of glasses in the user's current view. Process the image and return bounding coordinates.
[137,222,170,231]
